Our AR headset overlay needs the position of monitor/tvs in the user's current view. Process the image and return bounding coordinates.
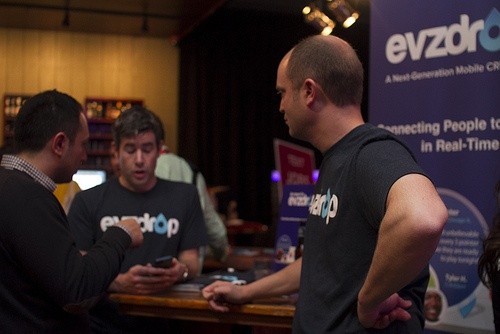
[71,169,107,190]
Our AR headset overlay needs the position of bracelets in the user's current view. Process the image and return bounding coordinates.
[183,267,188,279]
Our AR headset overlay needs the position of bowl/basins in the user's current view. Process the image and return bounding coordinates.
[225,224,268,244]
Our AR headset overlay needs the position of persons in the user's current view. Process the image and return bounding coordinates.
[155,152,232,265]
[0,89,143,334]
[67,103,202,295]
[202,34,448,334]
[424,292,442,322]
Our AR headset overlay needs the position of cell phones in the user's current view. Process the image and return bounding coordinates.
[153,255,173,269]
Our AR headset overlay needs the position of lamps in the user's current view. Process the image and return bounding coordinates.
[302,0,361,35]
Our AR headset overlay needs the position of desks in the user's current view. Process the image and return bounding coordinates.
[110,285,296,334]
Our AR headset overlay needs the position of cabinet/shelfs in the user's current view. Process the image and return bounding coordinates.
[85,99,143,155]
